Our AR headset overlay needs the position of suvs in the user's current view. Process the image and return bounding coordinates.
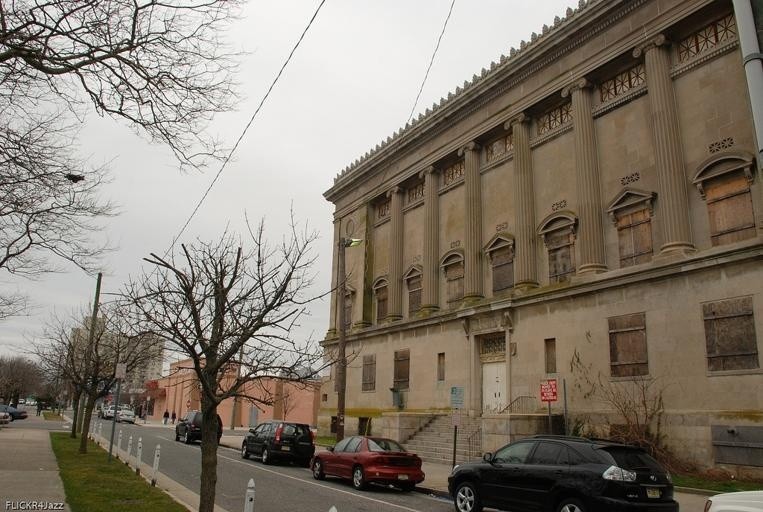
[240,420,316,468]
[174,409,223,445]
[446,433,681,512]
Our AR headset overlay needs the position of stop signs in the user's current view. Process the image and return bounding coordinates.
[186,400,191,408]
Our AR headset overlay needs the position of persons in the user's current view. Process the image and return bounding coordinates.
[34,399,41,416]
[161,408,169,425]
[170,409,176,424]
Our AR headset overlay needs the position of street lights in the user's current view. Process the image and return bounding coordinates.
[144,395,150,424]
[335,237,365,443]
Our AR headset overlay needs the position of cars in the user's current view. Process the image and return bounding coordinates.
[309,435,426,493]
[0,398,38,424]
[98,403,137,425]
[702,488,763,512]
